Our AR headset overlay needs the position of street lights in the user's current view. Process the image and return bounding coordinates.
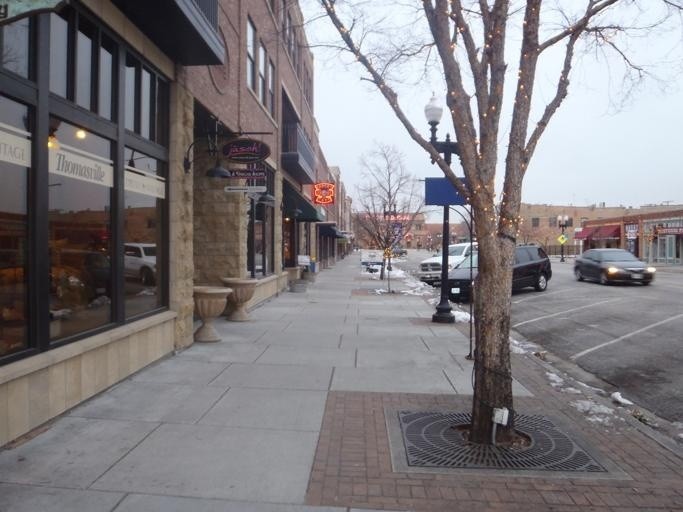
[423,91,465,324]
[556,215,569,263]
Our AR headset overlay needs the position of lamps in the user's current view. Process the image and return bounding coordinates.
[184,138,232,178]
[246,177,276,201]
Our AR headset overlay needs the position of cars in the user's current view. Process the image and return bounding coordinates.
[573,247,656,286]
[393,245,408,255]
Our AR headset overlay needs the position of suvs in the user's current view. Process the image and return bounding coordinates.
[417,241,480,288]
[123,240,159,287]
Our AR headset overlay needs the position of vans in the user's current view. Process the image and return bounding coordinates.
[447,244,553,304]
[59,248,112,296]
